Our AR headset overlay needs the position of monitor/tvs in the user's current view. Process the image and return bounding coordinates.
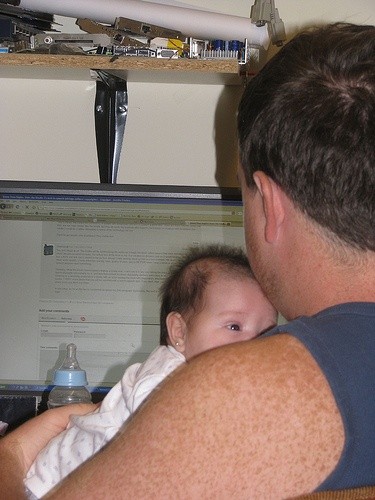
[0,179,248,429]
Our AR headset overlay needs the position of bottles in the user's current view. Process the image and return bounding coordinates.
[47,343,93,410]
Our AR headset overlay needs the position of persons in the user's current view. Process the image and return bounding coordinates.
[0,21,375,500]
